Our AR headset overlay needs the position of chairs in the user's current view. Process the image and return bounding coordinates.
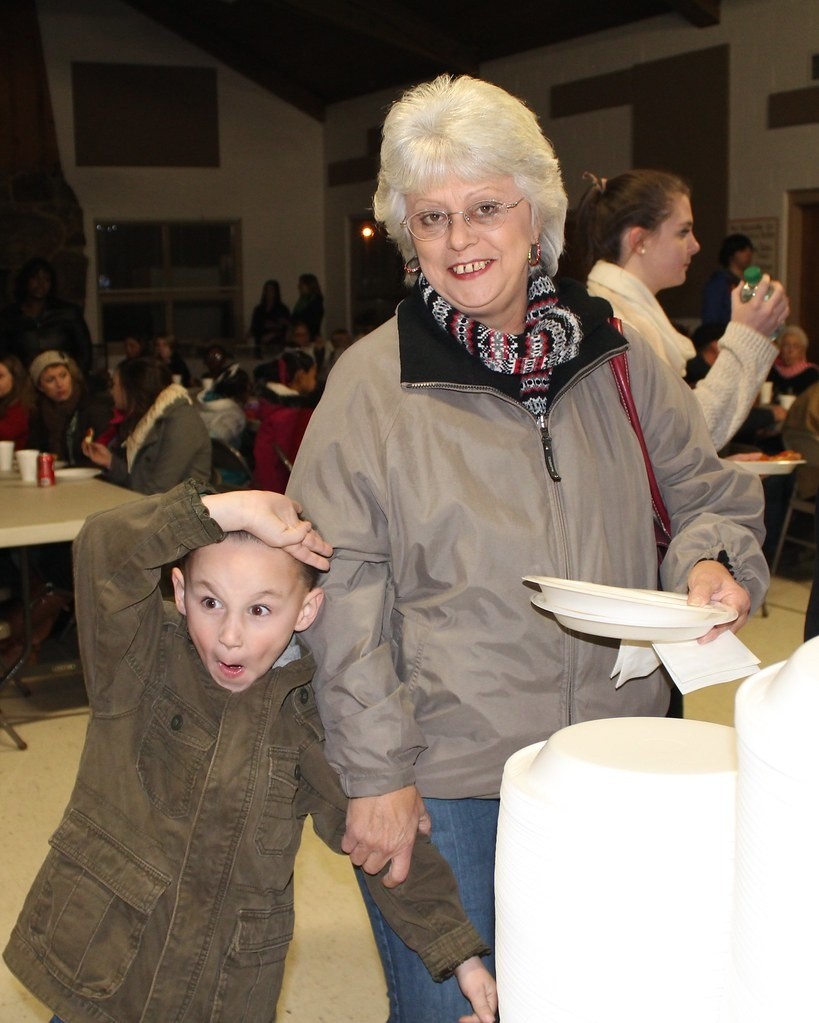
[725,407,819,617]
[213,436,294,491]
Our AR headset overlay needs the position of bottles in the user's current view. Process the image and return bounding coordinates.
[740,265,786,341]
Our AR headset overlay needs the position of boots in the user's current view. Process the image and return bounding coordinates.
[0,584,66,678]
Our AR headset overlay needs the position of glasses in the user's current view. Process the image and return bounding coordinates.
[399,197,527,242]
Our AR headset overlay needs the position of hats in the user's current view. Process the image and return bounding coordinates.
[29,350,68,387]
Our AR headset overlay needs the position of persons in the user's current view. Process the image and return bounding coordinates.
[0,272,351,677]
[579,173,789,719]
[686,236,819,564]
[0,480,496,1023]
[287,77,770,1023]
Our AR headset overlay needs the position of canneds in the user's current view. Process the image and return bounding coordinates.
[36,453,56,486]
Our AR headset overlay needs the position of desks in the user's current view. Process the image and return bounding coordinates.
[0,463,147,691]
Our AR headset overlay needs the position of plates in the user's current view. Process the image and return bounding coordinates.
[519,575,739,644]
[54,468,102,481]
[733,460,807,475]
[493,715,735,1023]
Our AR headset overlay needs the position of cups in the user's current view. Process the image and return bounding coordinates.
[14,449,39,482]
[0,441,14,471]
[202,378,213,389]
[779,395,796,410]
[759,381,773,404]
[172,374,182,384]
